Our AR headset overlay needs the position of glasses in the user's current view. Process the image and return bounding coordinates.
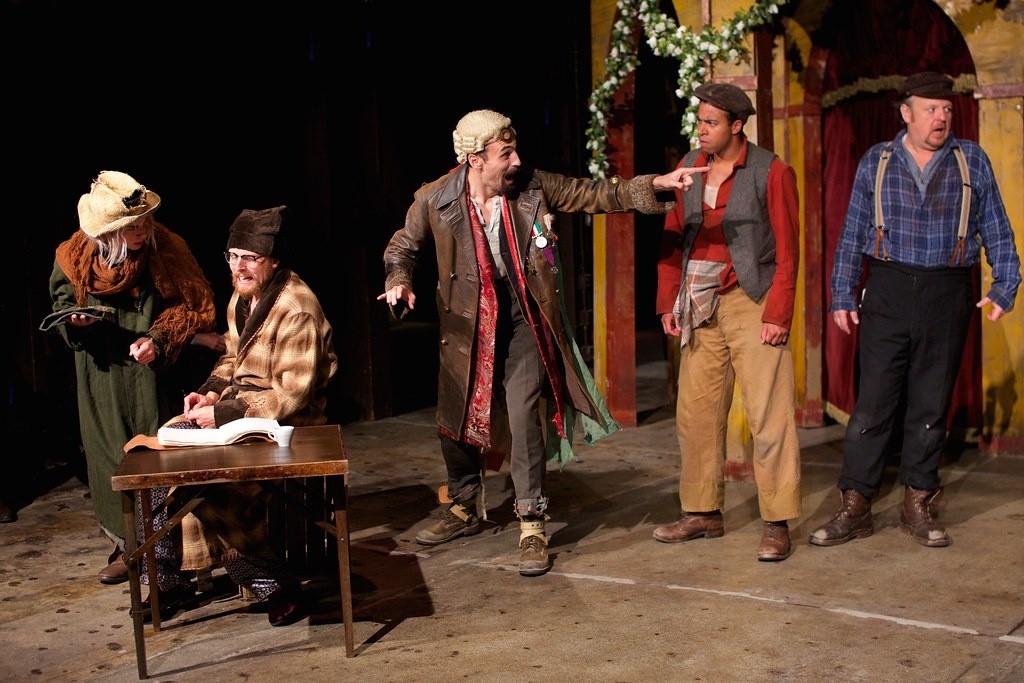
[224,251,266,265]
[485,126,518,147]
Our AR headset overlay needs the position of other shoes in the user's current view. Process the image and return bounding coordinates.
[130,582,197,622]
[98,539,144,584]
[0,493,18,523]
[268,575,308,626]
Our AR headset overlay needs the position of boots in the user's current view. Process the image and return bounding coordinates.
[416,496,481,545]
[516,512,550,575]
[899,484,949,546]
[809,488,874,545]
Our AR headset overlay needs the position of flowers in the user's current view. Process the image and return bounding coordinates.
[584,0,788,179]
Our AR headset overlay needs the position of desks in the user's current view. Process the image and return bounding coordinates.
[111,425,354,679]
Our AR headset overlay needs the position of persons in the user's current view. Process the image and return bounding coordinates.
[377,110,711,577]
[652,80,803,563]
[49,168,226,580]
[127,205,335,628]
[812,70,1021,550]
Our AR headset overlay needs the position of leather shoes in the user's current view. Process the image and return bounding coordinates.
[653,509,724,543]
[758,521,791,559]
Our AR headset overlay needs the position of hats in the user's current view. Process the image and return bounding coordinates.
[904,72,963,99]
[77,170,161,238]
[693,83,756,125]
[226,205,287,259]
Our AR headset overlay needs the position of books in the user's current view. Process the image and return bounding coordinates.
[157,416,281,448]
